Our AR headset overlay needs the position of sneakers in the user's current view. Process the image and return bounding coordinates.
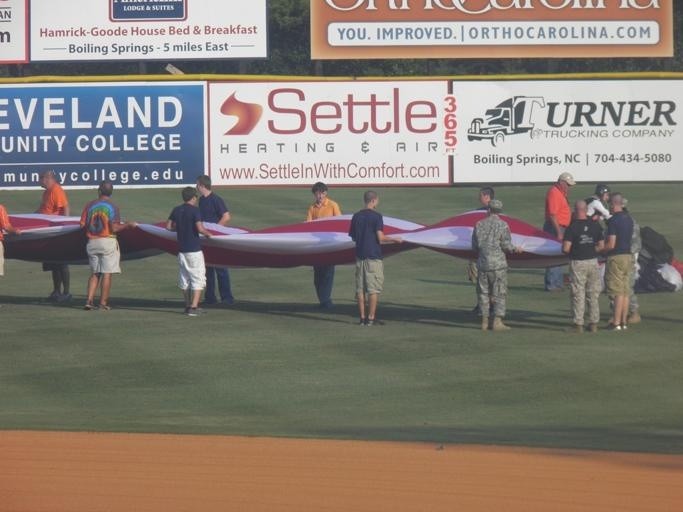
[576,311,642,333]
[47,291,71,302]
[85,300,111,311]
[183,307,206,317]
[359,317,384,327]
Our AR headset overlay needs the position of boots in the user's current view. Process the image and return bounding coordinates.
[481,316,488,330]
[492,317,510,331]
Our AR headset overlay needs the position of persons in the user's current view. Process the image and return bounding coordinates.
[79,180,136,310]
[0,204,20,276]
[195,175,235,307]
[562,184,643,333]
[33,170,73,304]
[471,199,524,329]
[306,181,342,308]
[166,186,213,317]
[468,188,494,319]
[348,190,403,327]
[543,171,576,291]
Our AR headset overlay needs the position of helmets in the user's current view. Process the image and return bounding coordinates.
[595,184,610,196]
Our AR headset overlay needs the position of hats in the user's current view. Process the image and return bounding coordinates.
[487,199,504,212]
[558,173,576,186]
